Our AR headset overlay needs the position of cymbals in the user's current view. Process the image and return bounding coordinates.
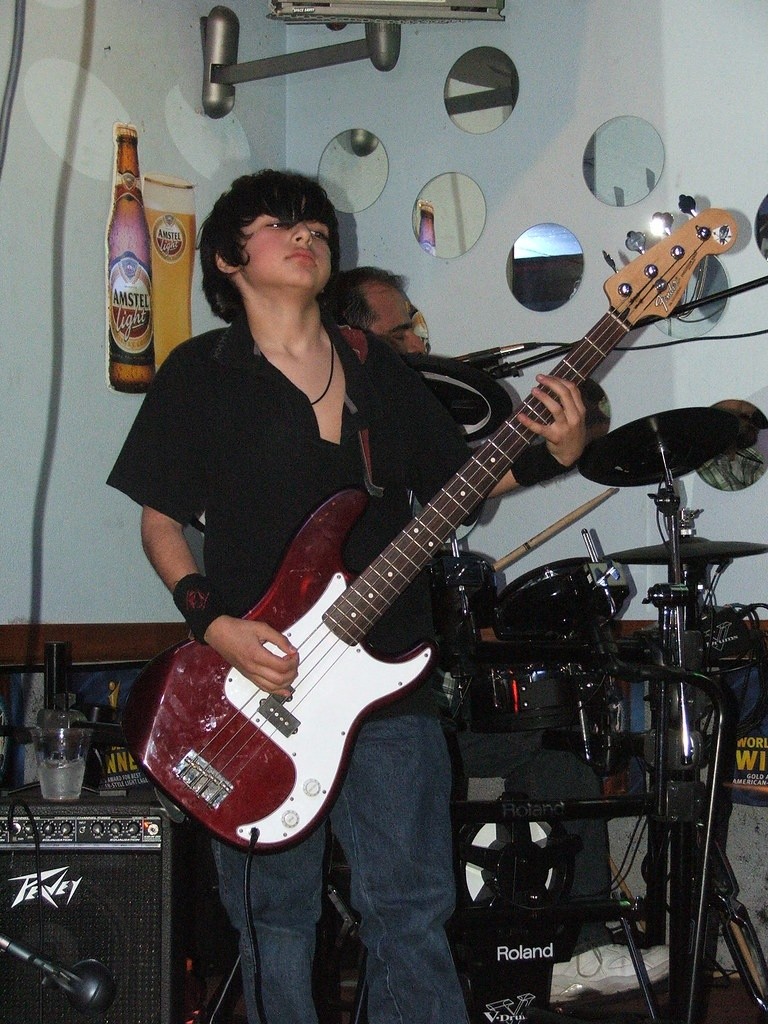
[607,534,768,563]
[382,352,513,443]
[577,407,737,488]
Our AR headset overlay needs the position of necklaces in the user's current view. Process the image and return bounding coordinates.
[310,332,337,406]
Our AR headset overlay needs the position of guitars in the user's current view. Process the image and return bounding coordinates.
[120,192,738,854]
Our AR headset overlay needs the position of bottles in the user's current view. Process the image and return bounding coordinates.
[420,203,436,252]
[106,125,155,394]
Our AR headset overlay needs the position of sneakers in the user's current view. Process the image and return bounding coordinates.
[549,941,670,1004]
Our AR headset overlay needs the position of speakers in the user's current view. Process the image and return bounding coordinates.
[0,779,238,1024]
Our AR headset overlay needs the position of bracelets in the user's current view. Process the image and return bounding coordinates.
[171,571,230,647]
[511,441,580,489]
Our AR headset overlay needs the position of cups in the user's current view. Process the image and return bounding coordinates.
[30,727,92,802]
[143,174,197,370]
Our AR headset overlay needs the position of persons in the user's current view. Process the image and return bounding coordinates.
[316,266,671,1004]
[105,168,587,1024]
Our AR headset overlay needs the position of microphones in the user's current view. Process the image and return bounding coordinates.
[30,959,117,1017]
[454,342,539,369]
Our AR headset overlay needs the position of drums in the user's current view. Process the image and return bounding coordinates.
[449,794,578,918]
[465,643,613,735]
[493,556,625,637]
[432,550,499,630]
